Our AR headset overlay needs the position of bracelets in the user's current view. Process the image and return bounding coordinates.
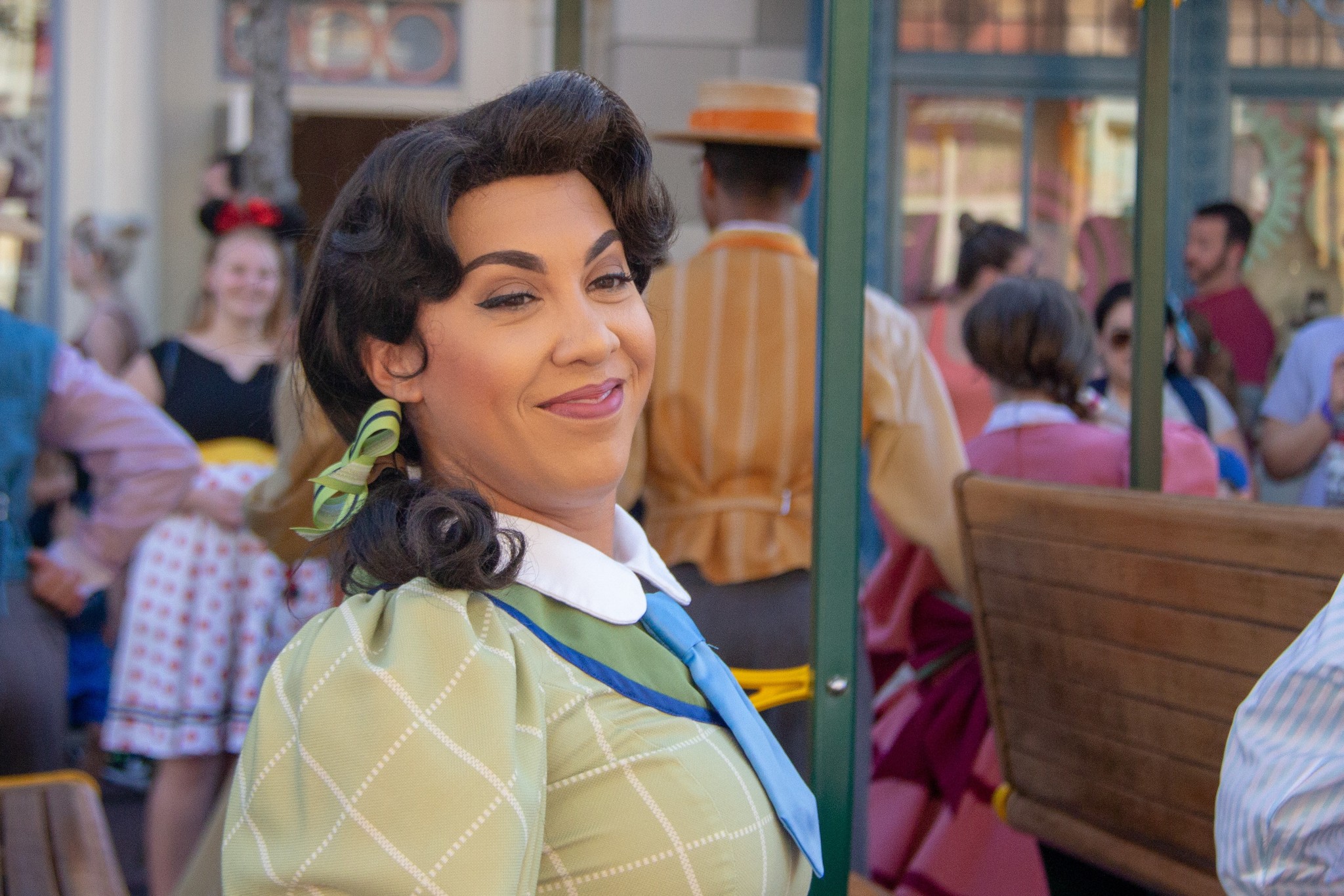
[1321,402,1334,424]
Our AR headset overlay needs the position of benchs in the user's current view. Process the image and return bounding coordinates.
[951,469,1344,896]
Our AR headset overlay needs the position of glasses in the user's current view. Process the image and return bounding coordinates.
[1102,329,1132,348]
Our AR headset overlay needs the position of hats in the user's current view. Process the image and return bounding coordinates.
[649,78,823,148]
[0,158,42,241]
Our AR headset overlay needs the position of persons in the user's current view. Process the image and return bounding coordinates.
[220,69,826,896]
[0,312,199,777]
[38,217,147,784]
[1257,314,1344,507]
[1079,280,1254,501]
[1214,548,1344,896]
[82,197,334,896]
[961,273,1220,498]
[1189,202,1278,397]
[909,215,1035,437]
[613,79,968,877]
